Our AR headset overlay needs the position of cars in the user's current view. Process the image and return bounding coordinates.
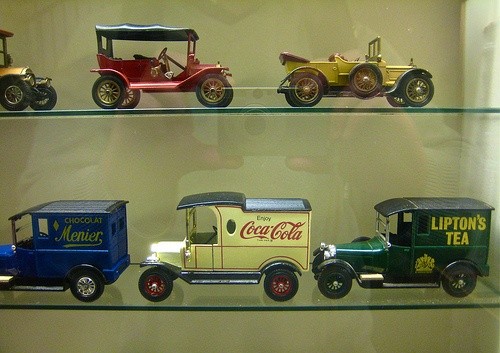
[276,35,435,108]
[90,22,234,108]
[0,28,58,112]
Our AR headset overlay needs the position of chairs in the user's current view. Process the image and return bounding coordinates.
[328,54,348,62]
[133,54,156,60]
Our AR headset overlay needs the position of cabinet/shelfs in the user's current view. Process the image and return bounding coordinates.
[0,1,500,353]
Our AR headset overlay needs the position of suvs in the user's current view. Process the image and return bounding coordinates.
[136,191,313,302]
[311,196,496,300]
[0,199,132,303]
